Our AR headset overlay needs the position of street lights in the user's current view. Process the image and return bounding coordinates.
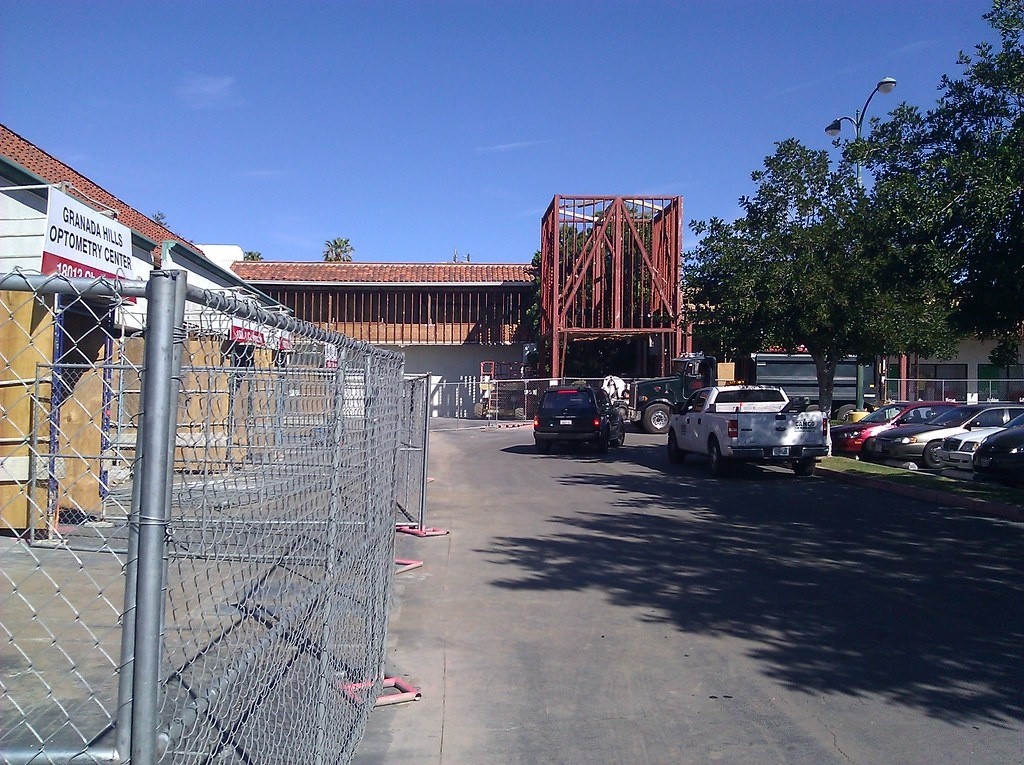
[825,77,898,408]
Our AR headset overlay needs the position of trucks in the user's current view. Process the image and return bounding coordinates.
[626,346,884,433]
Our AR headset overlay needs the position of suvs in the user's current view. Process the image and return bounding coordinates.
[533,383,626,450]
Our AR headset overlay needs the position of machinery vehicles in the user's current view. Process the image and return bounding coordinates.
[473,360,547,421]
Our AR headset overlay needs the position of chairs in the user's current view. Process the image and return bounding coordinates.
[715,393,730,403]
[582,393,593,408]
[901,409,910,419]
[907,410,921,423]
[922,410,931,423]
[551,396,571,408]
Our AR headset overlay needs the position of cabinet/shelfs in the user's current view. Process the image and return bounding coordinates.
[479,361,535,420]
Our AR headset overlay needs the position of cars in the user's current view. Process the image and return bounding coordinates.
[938,412,1024,471]
[972,423,1024,488]
[830,401,1024,471]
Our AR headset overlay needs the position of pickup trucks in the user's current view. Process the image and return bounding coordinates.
[667,380,830,477]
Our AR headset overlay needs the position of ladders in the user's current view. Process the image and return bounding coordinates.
[780,396,810,413]
